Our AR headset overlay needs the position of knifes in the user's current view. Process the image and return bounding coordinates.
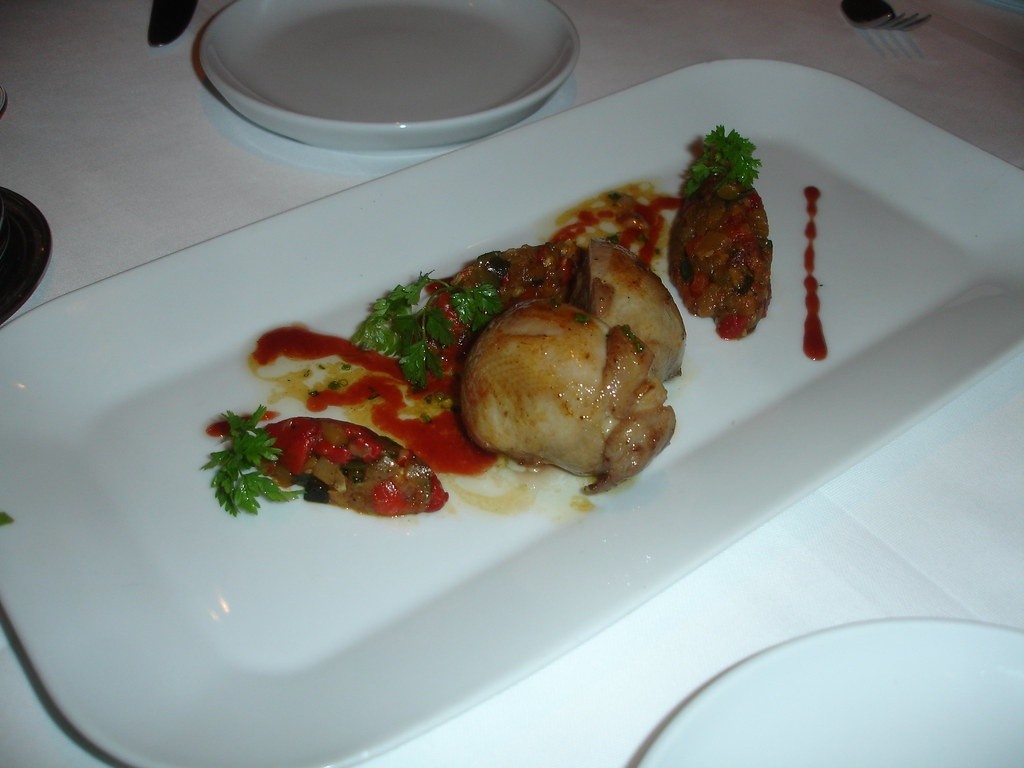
[148,0,195,47]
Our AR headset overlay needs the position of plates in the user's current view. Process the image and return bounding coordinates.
[198,1,581,151]
[628,616,1023,768]
[0,61,1024,768]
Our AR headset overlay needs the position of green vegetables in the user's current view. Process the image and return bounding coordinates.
[199,266,504,516]
[685,125,763,195]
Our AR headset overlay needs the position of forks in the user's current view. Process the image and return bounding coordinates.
[839,0,932,32]
